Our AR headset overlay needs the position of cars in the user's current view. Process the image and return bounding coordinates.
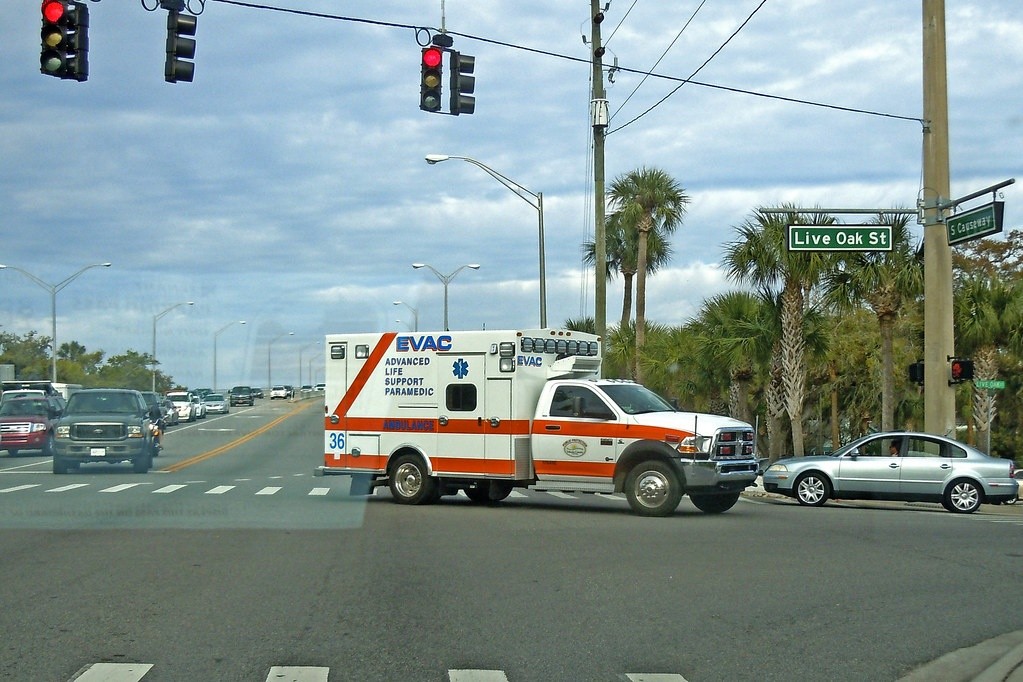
[51,389,152,474]
[314,383,326,392]
[763,432,1019,515]
[0,380,213,456]
[1003,469,1023,505]
[269,385,294,400]
[252,388,264,399]
[299,385,314,392]
[230,387,255,407]
[205,394,229,414]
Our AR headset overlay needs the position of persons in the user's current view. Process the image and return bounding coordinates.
[890,441,901,457]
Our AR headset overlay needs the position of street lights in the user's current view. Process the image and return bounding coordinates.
[300,340,320,388]
[268,332,295,388]
[426,155,547,329]
[214,320,247,393]
[152,301,194,393]
[0,263,112,383]
[393,301,417,332]
[412,263,481,331]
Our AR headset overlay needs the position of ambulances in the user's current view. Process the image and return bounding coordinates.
[313,327,760,517]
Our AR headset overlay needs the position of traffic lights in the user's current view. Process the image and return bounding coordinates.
[164,9,197,83]
[40,0,67,78]
[450,51,475,116]
[419,48,443,112]
[952,360,974,380]
[909,364,924,382]
